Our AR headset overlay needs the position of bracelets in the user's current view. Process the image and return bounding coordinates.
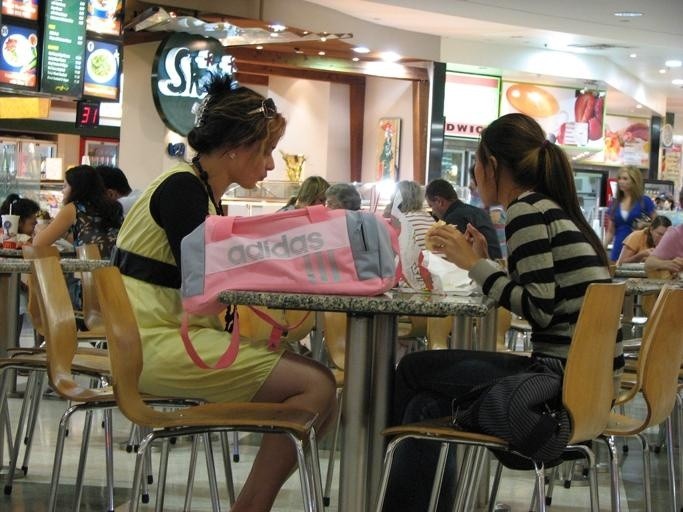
[65,232,70,240]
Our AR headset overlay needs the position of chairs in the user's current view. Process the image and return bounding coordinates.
[0,240,681,511]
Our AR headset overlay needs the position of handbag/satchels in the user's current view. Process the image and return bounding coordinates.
[450,355,572,465]
[178,203,403,371]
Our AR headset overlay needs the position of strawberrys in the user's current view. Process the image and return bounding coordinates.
[556,93,605,146]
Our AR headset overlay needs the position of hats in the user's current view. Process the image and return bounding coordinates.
[654,191,668,201]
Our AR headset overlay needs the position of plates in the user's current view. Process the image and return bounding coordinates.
[2,33,32,67]
[85,47,119,81]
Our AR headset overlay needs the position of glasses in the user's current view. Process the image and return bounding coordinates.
[246,97,277,119]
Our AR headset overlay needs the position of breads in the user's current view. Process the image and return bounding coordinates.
[424,219,457,250]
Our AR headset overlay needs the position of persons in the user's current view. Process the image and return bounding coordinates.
[79,166,144,349]
[273,176,502,288]
[32,165,124,401]
[112,74,338,512]
[604,165,683,275]
[383,113,624,512]
[0,194,40,375]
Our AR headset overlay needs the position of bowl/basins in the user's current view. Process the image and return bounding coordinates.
[28,33,38,46]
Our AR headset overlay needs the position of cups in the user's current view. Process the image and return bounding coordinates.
[0,214,20,248]
[503,82,568,130]
[90,1,117,30]
